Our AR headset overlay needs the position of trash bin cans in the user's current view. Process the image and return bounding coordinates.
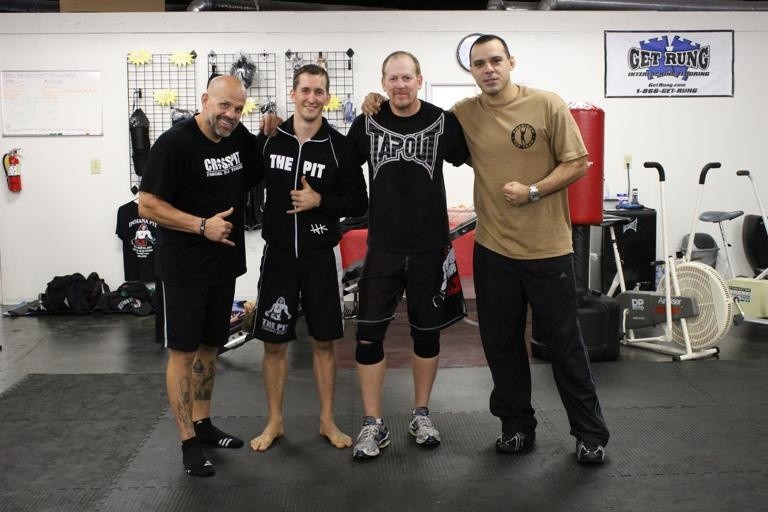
[680,234,720,271]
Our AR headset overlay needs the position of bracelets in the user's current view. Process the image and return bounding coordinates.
[200,218,206,239]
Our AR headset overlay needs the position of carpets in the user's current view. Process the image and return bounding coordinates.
[0,370,182,511]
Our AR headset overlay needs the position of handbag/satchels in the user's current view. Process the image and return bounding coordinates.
[42,272,109,315]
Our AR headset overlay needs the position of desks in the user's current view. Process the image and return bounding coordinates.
[342,208,477,330]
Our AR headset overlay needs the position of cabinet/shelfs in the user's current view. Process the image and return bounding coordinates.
[588,199,657,298]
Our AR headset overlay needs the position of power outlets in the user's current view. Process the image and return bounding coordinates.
[623,154,632,169]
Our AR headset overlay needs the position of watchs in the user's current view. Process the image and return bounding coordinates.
[530,183,540,203]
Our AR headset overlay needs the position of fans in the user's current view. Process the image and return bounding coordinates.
[651,259,736,361]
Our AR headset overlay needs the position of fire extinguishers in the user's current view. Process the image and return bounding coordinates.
[3,148,22,192]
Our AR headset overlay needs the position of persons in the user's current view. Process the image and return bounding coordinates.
[360,35,610,462]
[138,75,284,476]
[339,52,475,461]
[251,65,367,450]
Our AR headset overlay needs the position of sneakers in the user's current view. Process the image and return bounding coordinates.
[352,415,391,459]
[180,436,215,478]
[192,416,246,450]
[575,439,606,465]
[408,407,441,447]
[497,430,536,454]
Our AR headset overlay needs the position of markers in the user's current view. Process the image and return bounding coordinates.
[49,133,62,136]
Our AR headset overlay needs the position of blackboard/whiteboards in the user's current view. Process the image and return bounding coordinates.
[0,70,104,136]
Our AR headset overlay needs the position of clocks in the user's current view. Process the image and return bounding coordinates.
[455,33,484,73]
